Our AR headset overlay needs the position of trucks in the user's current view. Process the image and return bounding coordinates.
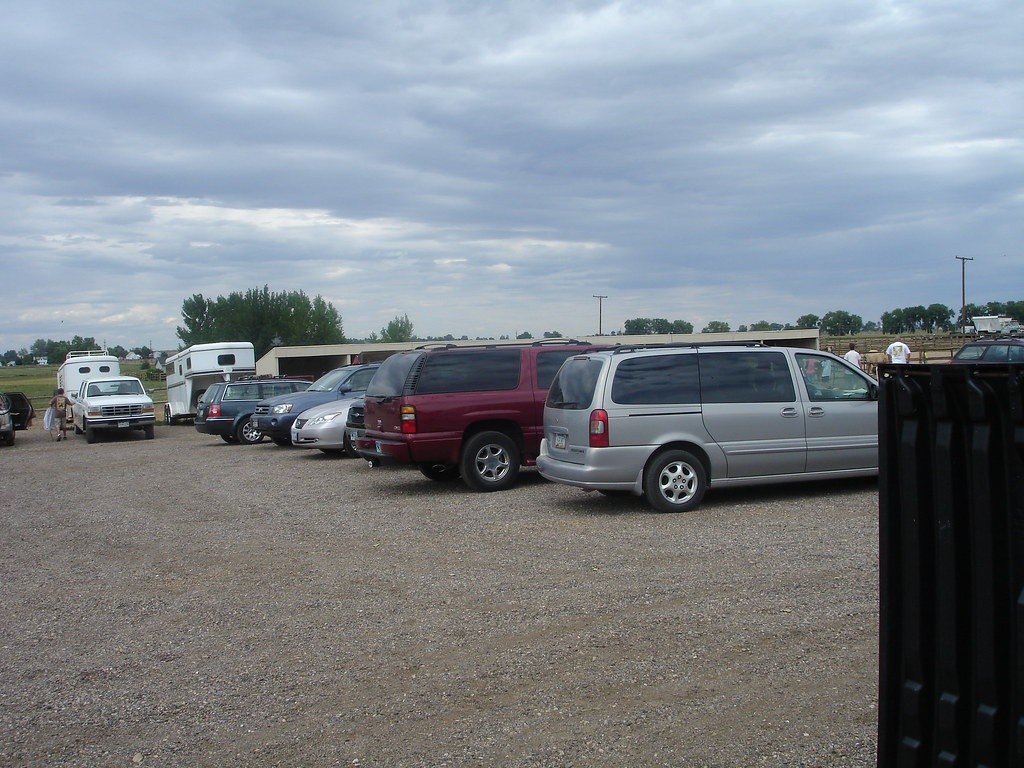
[163,342,254,425]
[57,350,121,423]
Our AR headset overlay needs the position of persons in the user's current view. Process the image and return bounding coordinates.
[817,358,831,383]
[885,334,911,364]
[805,358,820,385]
[843,342,862,389]
[49,387,76,441]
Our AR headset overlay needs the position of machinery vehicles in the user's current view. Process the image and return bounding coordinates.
[970,316,1024,340]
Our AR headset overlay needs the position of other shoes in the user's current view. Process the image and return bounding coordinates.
[63,436,67,440]
[57,434,61,441]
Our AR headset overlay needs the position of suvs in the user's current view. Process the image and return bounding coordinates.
[250,361,383,446]
[950,334,1024,364]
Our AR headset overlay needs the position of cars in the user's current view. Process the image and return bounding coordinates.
[290,394,367,457]
[0,391,36,446]
[71,376,156,443]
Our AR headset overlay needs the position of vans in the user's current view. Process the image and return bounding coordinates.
[355,339,633,491]
[194,375,331,444]
[535,341,878,512]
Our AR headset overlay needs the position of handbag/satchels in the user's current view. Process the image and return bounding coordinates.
[54,408,66,418]
